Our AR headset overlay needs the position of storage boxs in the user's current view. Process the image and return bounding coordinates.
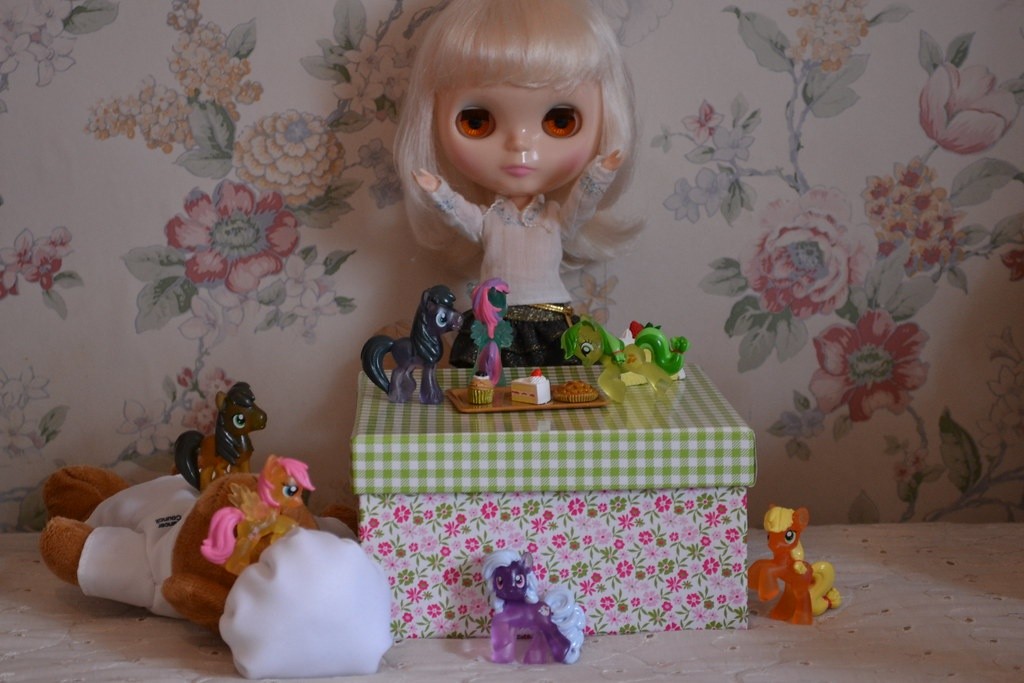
[348,363,759,642]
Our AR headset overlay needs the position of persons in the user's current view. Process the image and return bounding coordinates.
[393,0,636,363]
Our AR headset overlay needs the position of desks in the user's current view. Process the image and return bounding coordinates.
[0,520,1024,683]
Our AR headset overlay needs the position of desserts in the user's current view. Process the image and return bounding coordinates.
[511,368,551,404]
[552,380,599,402]
[468,370,494,404]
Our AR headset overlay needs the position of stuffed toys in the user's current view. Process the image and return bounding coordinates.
[41,463,394,678]
